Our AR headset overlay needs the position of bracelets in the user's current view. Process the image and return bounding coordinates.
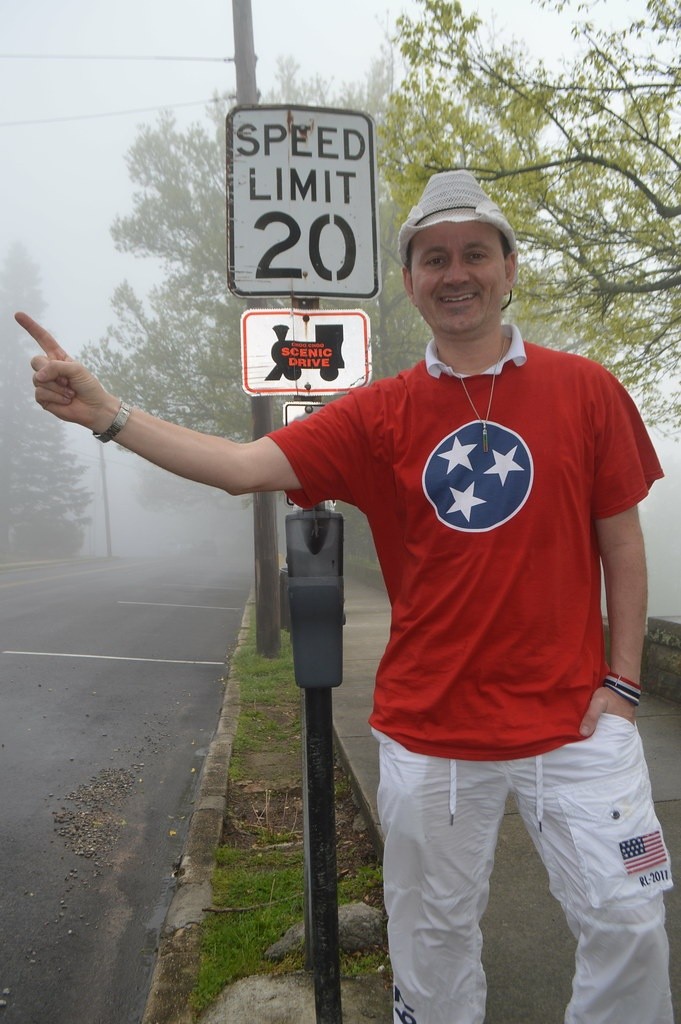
[92,401,131,443]
[604,672,642,706]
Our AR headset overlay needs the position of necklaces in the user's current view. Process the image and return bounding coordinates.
[436,338,506,452]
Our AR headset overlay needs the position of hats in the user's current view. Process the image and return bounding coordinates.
[399,170,519,265]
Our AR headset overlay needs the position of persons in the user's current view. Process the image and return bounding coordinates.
[14,169,673,1024]
